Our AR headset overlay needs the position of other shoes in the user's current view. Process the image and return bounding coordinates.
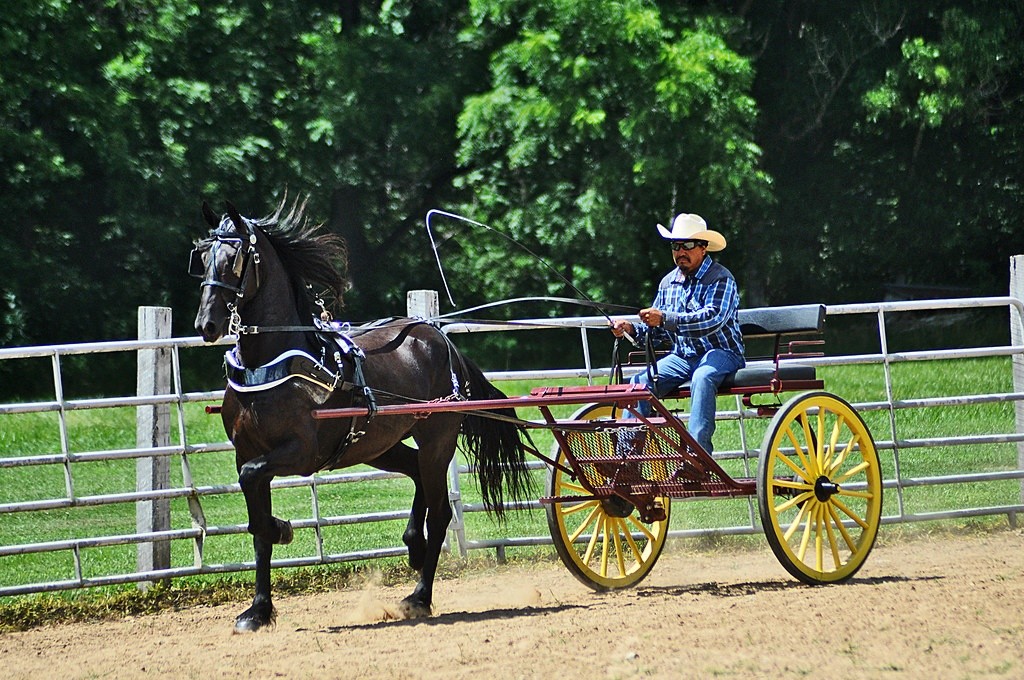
[594,462,643,483]
[674,462,711,482]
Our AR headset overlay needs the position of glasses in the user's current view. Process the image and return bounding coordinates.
[670,241,702,251]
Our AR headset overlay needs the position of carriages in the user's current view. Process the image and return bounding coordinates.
[191,182,885,635]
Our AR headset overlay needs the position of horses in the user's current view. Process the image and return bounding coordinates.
[191,185,543,635]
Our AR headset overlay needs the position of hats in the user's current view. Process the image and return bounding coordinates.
[657,213,727,251]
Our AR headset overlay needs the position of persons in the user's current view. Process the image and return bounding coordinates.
[594,213,748,487]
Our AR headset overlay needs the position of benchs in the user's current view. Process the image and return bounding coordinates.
[610,305,827,421]
